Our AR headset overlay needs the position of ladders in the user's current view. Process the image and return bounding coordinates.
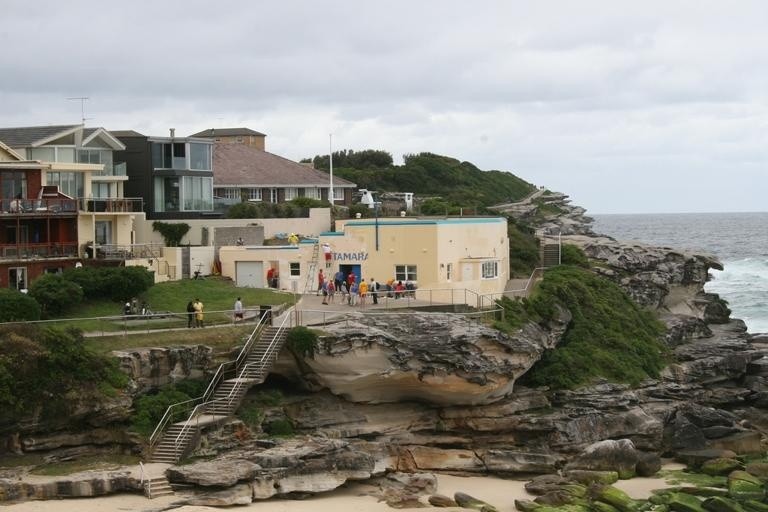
[304,245,318,294]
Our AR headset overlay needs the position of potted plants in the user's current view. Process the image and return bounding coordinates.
[350,205,368,219]
[399,208,407,218]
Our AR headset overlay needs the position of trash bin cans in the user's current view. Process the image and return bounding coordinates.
[260,306,272,326]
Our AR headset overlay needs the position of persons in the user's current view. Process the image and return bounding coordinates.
[234,297,247,325]
[340,280,349,304]
[186,300,197,328]
[123,297,152,320]
[346,270,356,293]
[348,276,418,307]
[321,280,328,304]
[267,268,275,288]
[320,242,334,268]
[193,298,205,329]
[286,233,299,247]
[333,268,344,294]
[236,237,245,247]
[317,268,327,296]
[326,279,336,303]
